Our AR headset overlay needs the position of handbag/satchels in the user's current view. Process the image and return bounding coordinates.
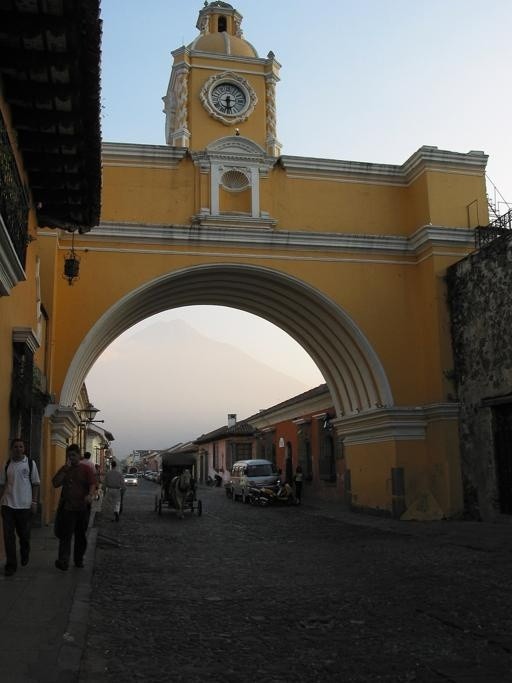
[54,511,62,538]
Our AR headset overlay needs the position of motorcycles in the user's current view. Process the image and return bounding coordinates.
[248,477,300,506]
[224,482,233,498]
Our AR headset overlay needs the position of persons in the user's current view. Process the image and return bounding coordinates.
[80,451,97,476]
[293,465,303,504]
[50,443,97,570]
[104,460,126,523]
[0,437,42,576]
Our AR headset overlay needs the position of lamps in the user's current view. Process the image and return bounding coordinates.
[61,231,80,286]
[82,402,99,421]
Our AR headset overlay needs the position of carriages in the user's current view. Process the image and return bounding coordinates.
[153,448,204,518]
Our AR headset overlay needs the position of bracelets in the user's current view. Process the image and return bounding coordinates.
[32,496,38,504]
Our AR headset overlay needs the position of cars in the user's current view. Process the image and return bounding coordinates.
[123,469,163,486]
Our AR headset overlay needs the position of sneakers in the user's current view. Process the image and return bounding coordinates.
[20,554,29,566]
[55,560,69,570]
[75,561,84,568]
[114,511,119,522]
[5,568,14,576]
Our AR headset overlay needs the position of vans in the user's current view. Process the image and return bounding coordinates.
[230,459,281,503]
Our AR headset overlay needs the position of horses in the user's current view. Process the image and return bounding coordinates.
[166,469,193,519]
